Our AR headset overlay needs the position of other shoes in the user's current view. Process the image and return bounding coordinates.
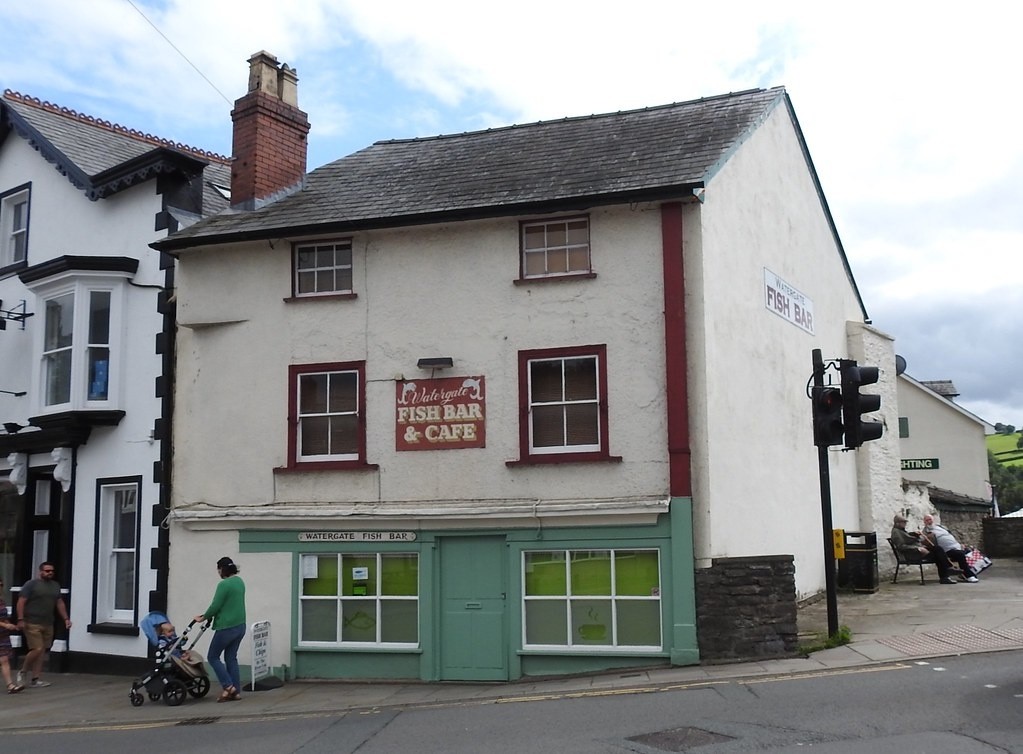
[940,575,957,584]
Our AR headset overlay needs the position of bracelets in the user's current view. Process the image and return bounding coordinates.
[17,618,24,622]
[64,617,69,621]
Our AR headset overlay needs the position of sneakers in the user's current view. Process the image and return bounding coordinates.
[17,671,27,687]
[961,572,980,583]
[29,677,53,687]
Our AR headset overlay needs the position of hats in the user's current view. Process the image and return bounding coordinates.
[894,515,909,523]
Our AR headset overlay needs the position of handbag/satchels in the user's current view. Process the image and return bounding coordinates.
[960,542,992,578]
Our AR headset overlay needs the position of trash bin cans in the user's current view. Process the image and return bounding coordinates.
[845,531,880,595]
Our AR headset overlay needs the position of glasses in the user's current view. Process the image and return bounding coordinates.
[41,569,54,573]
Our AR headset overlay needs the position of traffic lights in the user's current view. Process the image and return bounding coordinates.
[811,386,845,447]
[838,358,883,449]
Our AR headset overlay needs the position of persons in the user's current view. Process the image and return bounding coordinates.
[195,556,247,703]
[0,576,26,694]
[922,514,979,583]
[891,514,957,584]
[17,562,72,687]
[158,622,191,661]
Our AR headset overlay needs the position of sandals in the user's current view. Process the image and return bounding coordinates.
[214,685,240,702]
[6,682,25,695]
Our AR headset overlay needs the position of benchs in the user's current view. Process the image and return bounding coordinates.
[886,537,975,585]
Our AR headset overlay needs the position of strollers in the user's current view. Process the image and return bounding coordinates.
[127,611,212,707]
